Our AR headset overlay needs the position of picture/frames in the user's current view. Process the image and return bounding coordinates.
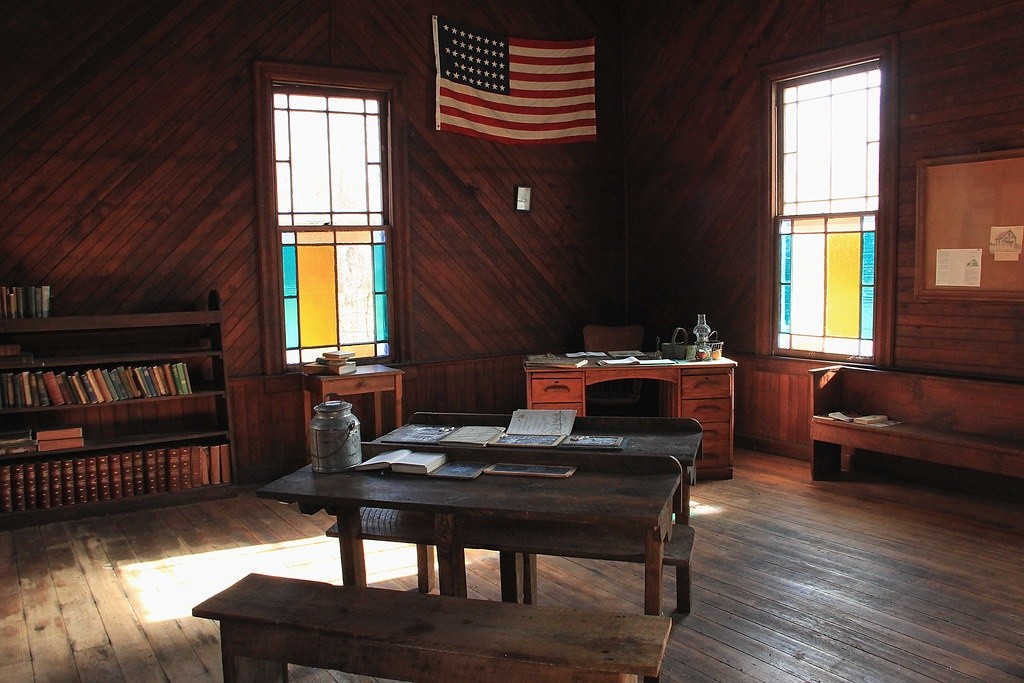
[916,147,1024,301]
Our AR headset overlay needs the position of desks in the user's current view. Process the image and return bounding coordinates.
[301,364,405,466]
[370,411,702,614]
[523,353,738,481]
[256,442,682,683]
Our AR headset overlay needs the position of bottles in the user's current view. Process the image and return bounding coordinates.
[693,313,712,362]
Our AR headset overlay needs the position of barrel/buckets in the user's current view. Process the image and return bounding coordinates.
[307,396,361,473]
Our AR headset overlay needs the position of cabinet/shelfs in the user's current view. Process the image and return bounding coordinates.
[0,289,239,533]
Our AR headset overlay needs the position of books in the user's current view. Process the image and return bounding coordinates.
[567,351,608,359]
[813,414,837,421]
[597,357,675,367]
[304,351,358,375]
[1,284,53,318]
[440,426,507,448]
[525,358,588,369]
[0,441,232,515]
[0,426,84,457]
[829,409,865,423]
[1,362,192,409]
[356,448,449,474]
[854,415,888,424]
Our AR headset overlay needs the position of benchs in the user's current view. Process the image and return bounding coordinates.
[193,573,672,683]
[807,365,1024,481]
[327,507,696,613]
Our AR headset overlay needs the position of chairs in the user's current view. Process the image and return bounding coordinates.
[583,325,645,415]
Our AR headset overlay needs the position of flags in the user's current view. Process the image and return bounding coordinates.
[432,16,598,145]
